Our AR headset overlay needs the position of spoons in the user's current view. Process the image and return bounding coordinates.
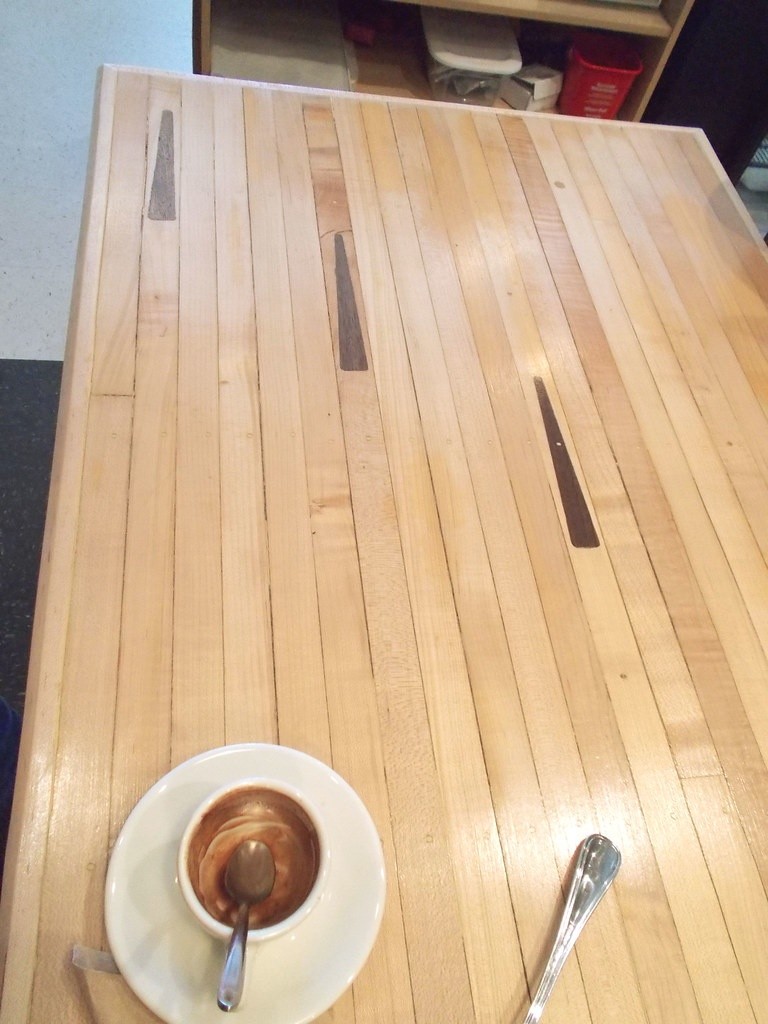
[217,840,276,1012]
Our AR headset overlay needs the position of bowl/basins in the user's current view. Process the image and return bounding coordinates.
[177,779,329,944]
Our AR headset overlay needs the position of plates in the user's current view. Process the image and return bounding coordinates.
[104,742,387,1024]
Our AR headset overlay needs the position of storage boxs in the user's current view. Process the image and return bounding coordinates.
[420,6,564,112]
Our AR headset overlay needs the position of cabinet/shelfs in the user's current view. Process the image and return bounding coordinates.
[195,0,694,123]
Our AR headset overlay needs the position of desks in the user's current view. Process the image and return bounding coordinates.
[0,61,768,1024]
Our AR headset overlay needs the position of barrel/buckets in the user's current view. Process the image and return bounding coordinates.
[558,30,644,120]
